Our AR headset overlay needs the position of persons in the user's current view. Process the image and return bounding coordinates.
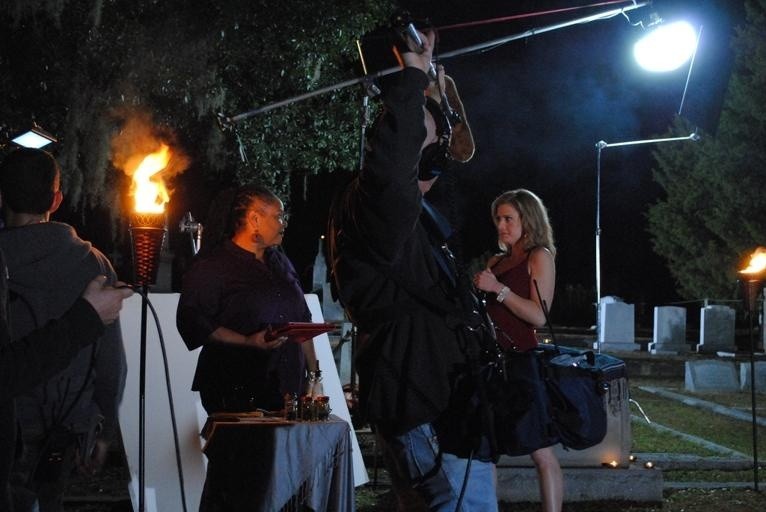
[3,148,133,512]
[330,29,495,512]
[473,189,565,512]
[53,185,133,274]
[177,186,324,416]
[2,274,132,512]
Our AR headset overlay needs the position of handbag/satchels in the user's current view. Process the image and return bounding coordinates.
[439,280,607,463]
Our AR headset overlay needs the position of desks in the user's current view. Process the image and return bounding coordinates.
[205,410,350,512]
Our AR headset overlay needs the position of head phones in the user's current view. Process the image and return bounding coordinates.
[417,96,452,182]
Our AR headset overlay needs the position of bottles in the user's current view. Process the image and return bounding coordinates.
[311,359,324,399]
[285,394,330,422]
[546,354,588,367]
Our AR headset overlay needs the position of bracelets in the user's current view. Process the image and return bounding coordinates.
[305,369,323,385]
[496,289,508,302]
